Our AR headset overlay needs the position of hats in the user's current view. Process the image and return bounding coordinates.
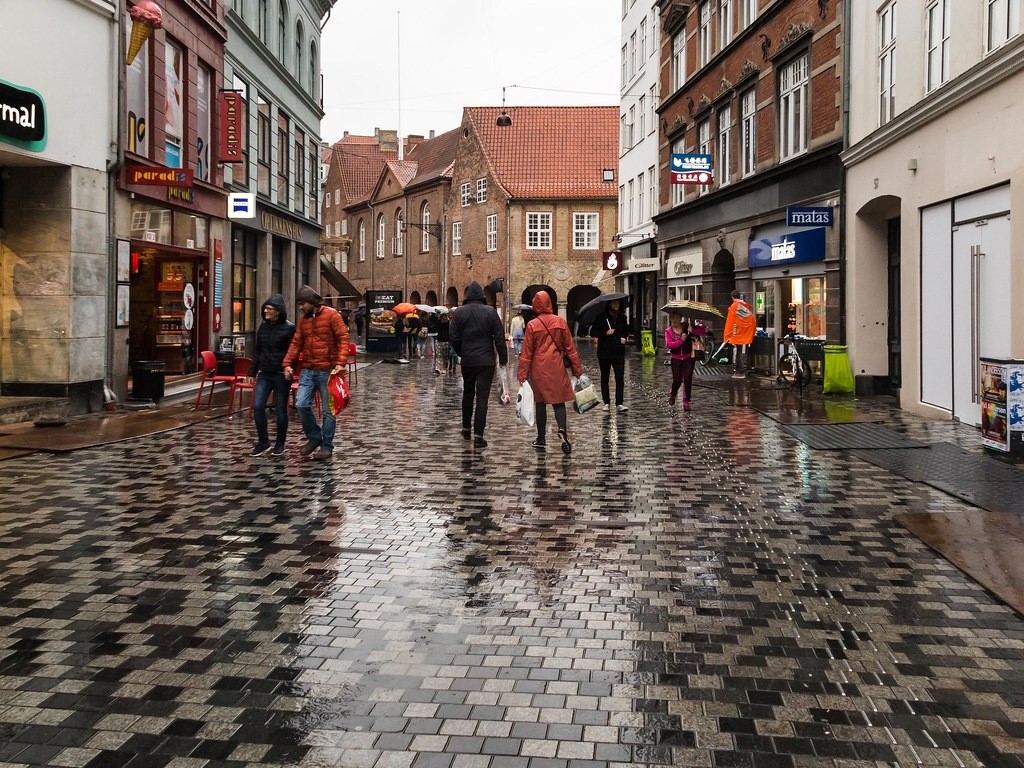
[296,285,323,309]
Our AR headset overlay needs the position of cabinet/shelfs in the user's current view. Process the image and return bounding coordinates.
[155,291,191,340]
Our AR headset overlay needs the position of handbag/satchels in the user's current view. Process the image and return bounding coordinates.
[326,368,354,417]
[497,365,512,406]
[570,374,601,414]
[561,352,572,369]
[509,338,515,349]
[515,380,536,428]
[691,334,707,362]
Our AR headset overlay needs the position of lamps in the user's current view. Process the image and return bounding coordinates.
[401,221,441,244]
[496,85,513,126]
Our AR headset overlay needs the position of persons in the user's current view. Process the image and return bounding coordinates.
[283,286,350,459]
[450,281,507,448]
[665,311,706,411]
[248,294,297,456]
[589,300,629,412]
[393,312,457,374]
[510,312,525,357]
[341,311,364,338]
[517,290,582,453]
[724,291,756,378]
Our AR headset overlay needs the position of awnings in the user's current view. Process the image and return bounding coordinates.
[321,255,362,301]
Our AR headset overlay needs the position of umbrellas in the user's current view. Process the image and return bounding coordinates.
[512,304,532,310]
[392,303,448,314]
[660,300,725,335]
[338,303,366,312]
[577,292,632,329]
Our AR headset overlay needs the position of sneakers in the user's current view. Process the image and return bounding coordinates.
[271,441,286,456]
[531,439,548,448]
[557,428,572,454]
[248,442,272,457]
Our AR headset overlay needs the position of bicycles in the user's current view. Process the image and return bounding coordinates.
[780,330,812,396]
[699,331,715,366]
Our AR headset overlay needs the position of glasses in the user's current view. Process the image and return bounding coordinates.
[264,307,274,312]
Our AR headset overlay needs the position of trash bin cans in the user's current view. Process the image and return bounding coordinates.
[131,361,165,401]
[215,351,236,376]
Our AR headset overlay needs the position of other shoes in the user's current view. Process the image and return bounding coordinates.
[300,440,322,456]
[453,368,456,374]
[602,404,610,411]
[473,437,488,447]
[449,370,453,376]
[669,392,675,405]
[461,429,472,440]
[732,372,747,379]
[417,349,421,357]
[420,355,427,359]
[442,370,447,374]
[435,368,441,375]
[683,400,694,410]
[615,405,629,412]
[311,446,336,460]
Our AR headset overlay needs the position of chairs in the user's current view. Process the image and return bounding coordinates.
[227,356,258,420]
[194,351,244,413]
[284,342,358,421]
